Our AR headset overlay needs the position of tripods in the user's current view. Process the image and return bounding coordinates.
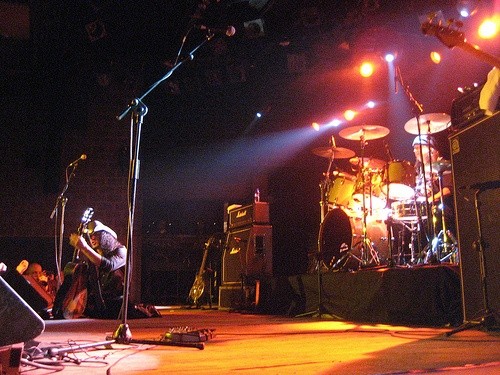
[332,84,459,272]
[443,189,500,337]
[45,38,204,356]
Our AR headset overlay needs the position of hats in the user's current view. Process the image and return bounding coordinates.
[87,220,118,241]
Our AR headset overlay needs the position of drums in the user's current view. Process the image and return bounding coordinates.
[318,208,394,273]
[325,170,361,216]
[351,168,386,209]
[391,202,427,220]
[381,160,418,201]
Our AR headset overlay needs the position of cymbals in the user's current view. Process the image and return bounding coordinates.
[404,112,452,135]
[311,146,356,158]
[349,157,388,171]
[339,125,390,140]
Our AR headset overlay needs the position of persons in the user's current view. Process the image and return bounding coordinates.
[26,219,127,299]
[411,135,453,238]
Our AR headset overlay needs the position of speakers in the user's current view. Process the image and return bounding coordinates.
[0,259,47,347]
[221,225,272,284]
[447,110,500,327]
[218,286,269,311]
[229,202,270,228]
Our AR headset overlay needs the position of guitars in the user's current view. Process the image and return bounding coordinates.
[188,237,214,300]
[419,12,500,71]
[52,207,95,319]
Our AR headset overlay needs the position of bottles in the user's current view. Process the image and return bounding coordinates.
[255,188,259,203]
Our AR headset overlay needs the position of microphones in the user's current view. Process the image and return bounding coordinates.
[194,24,235,36]
[385,143,396,163]
[70,154,87,166]
[394,66,398,94]
[470,178,500,190]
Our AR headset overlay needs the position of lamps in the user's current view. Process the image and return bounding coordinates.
[429,46,453,65]
